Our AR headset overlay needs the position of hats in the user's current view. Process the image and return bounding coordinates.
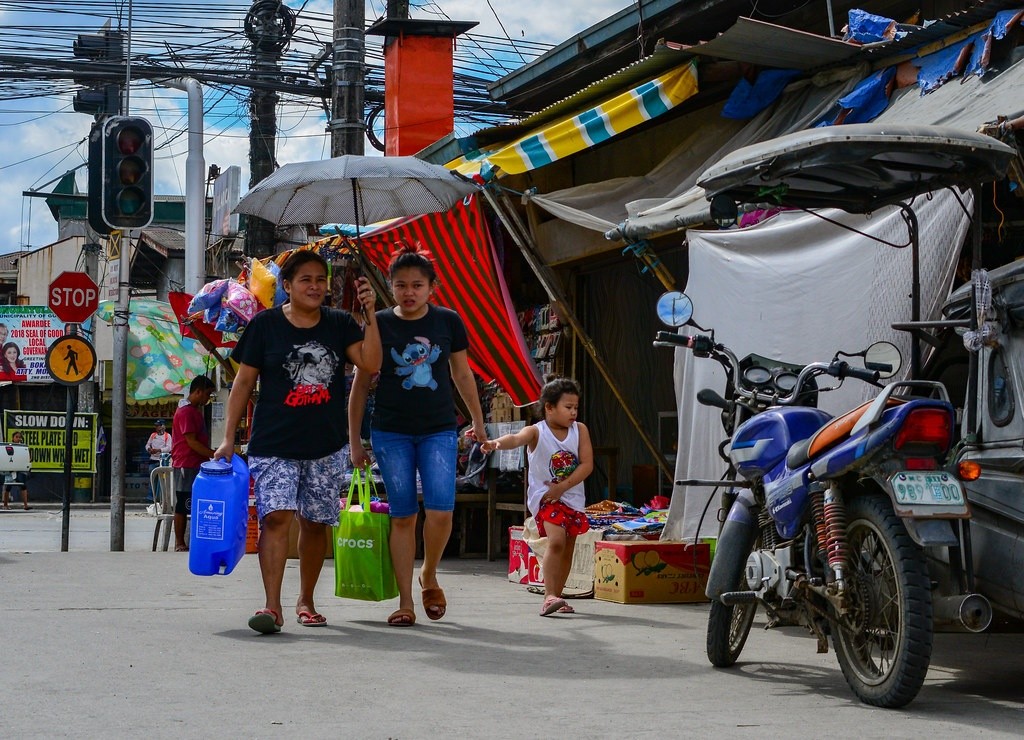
[154,418,166,426]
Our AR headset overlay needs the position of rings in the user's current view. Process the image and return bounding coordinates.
[370,291,372,296]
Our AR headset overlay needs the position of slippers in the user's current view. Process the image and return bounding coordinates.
[388,608,416,626]
[418,575,447,620]
[248,608,281,633]
[175,547,189,552]
[296,610,327,627]
[556,606,574,613]
[539,597,566,616]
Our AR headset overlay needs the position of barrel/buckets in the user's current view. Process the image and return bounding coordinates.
[681,536,717,562]
[189,453,251,574]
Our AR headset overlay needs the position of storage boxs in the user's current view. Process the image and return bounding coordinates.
[528,552,545,585]
[287,517,334,559]
[592,541,712,605]
[246,500,258,553]
[507,526,529,585]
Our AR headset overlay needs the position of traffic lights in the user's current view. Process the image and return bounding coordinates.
[99,114,156,232]
[71,29,123,121]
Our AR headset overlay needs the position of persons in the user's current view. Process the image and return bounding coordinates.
[211,247,382,632]
[0,342,27,382]
[169,374,215,551]
[347,238,492,629]
[0,323,11,368]
[479,373,595,617]
[3,432,32,510]
[143,420,172,504]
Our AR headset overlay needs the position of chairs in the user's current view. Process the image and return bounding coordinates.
[487,466,529,561]
[151,466,192,552]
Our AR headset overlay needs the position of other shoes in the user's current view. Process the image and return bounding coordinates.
[142,498,153,503]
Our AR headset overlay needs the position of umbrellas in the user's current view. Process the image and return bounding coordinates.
[229,154,482,316]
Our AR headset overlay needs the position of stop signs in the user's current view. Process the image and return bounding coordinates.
[46,270,100,325]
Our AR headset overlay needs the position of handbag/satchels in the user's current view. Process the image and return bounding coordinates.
[331,461,400,602]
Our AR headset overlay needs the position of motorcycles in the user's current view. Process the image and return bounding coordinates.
[650,290,994,709]
[888,256,1024,624]
